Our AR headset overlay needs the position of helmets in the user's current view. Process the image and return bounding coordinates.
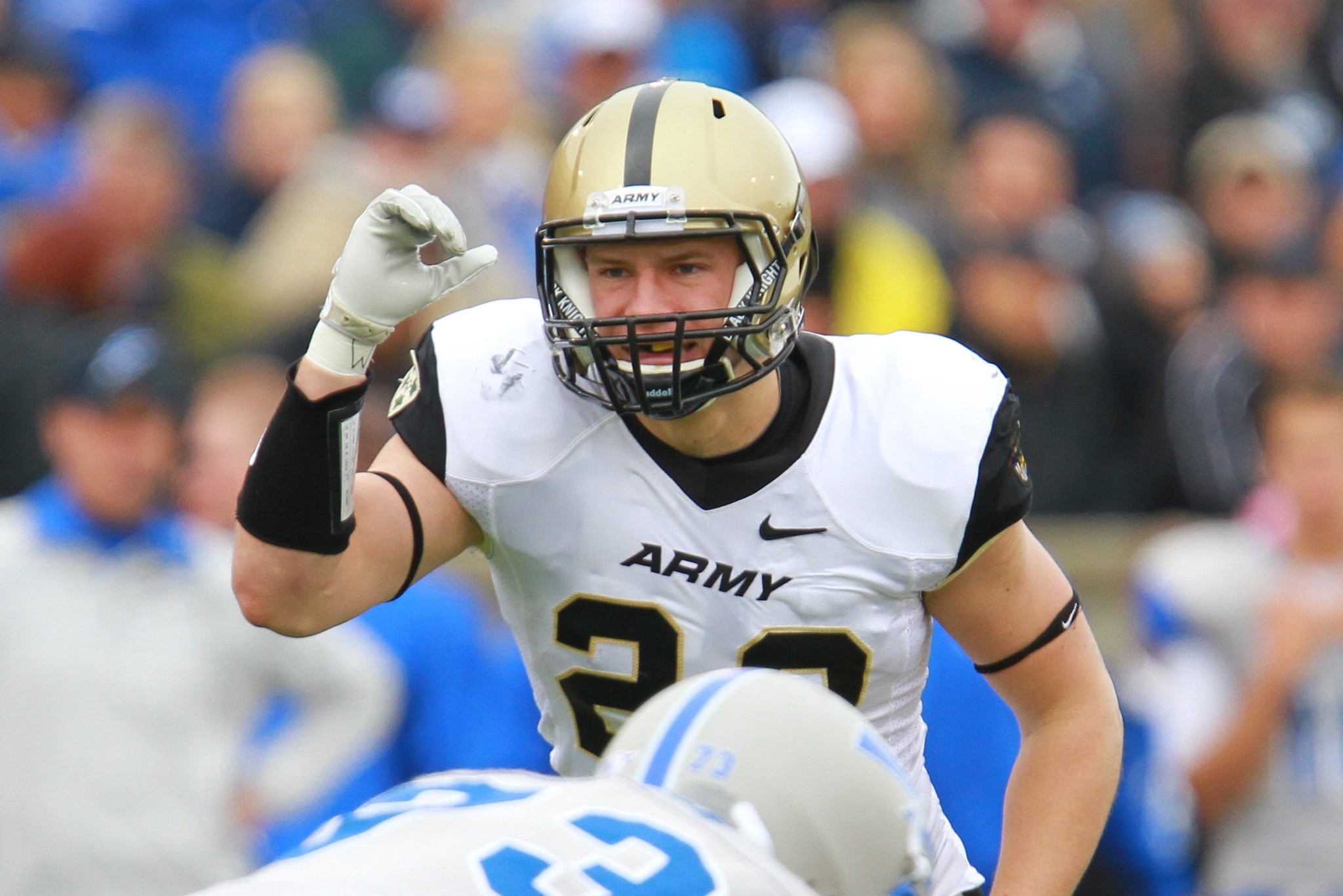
[742,81,870,185]
[534,77,818,423]
[594,668,940,896]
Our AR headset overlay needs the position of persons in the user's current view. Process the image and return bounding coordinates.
[231,73,1124,896]
[1,1,1343,896]
[189,667,923,896]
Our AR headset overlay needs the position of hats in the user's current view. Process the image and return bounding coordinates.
[29,307,193,406]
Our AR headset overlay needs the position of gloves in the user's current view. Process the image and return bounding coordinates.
[302,183,499,377]
